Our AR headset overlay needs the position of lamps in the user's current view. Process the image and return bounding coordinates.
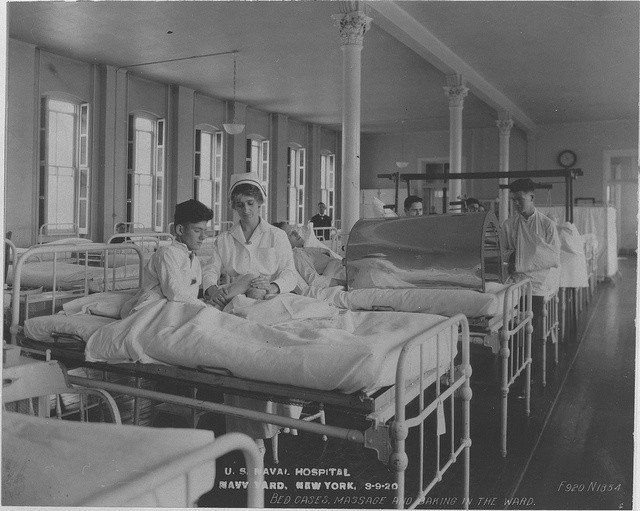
[396,119,410,170]
[221,50,246,136]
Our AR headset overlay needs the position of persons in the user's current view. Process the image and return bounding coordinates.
[202,171,297,465]
[466,197,479,212]
[310,202,331,240]
[128,199,266,311]
[498,178,559,400]
[403,195,424,217]
[111,223,134,244]
[271,221,346,288]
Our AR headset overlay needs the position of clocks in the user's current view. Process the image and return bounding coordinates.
[557,148,576,168]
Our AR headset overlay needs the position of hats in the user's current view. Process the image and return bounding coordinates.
[228,172,267,208]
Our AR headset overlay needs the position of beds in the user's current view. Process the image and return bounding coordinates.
[10,242,475,509]
[114,221,172,247]
[6,259,145,294]
[1,411,268,508]
[211,221,235,235]
[312,225,338,244]
[485,235,613,389]
[37,222,174,267]
[269,241,533,459]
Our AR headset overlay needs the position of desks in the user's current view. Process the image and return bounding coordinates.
[3,340,70,420]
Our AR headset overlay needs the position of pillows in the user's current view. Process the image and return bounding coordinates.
[289,222,343,262]
[61,290,134,320]
